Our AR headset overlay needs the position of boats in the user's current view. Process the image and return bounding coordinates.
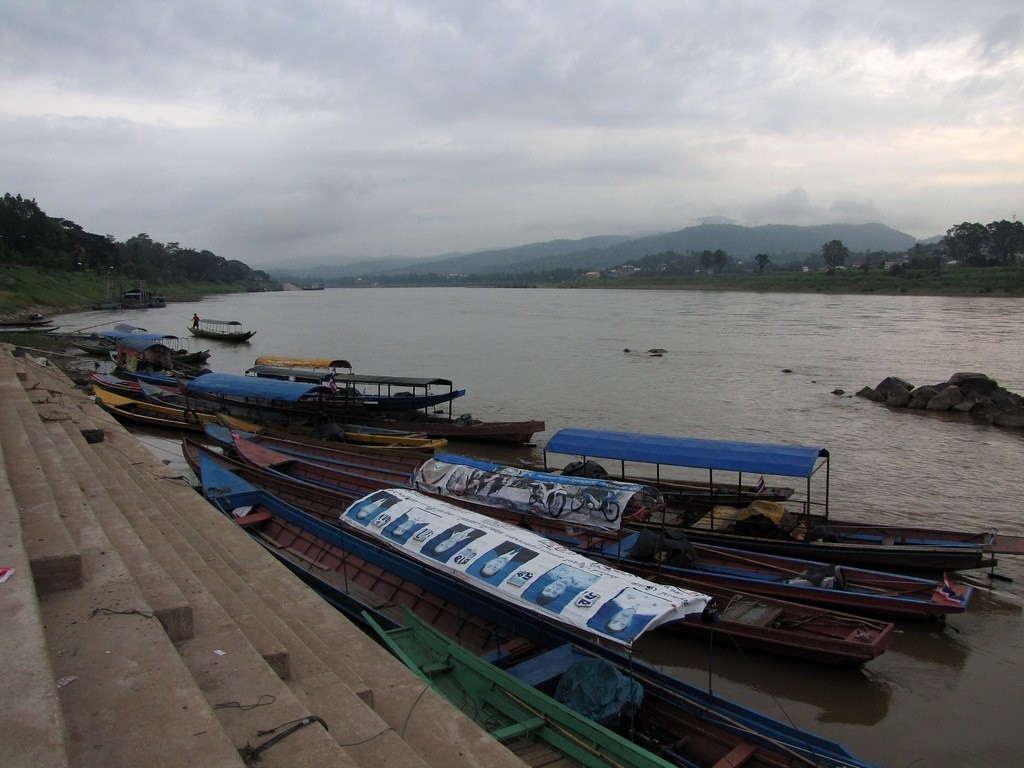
[92,303,122,309]
[151,300,167,307]
[0,324,62,333]
[71,324,1024,668]
[357,598,673,767]
[0,312,55,324]
[194,446,874,767]
[186,318,257,342]
[121,299,150,308]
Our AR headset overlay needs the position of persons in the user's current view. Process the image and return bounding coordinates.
[191,313,199,330]
